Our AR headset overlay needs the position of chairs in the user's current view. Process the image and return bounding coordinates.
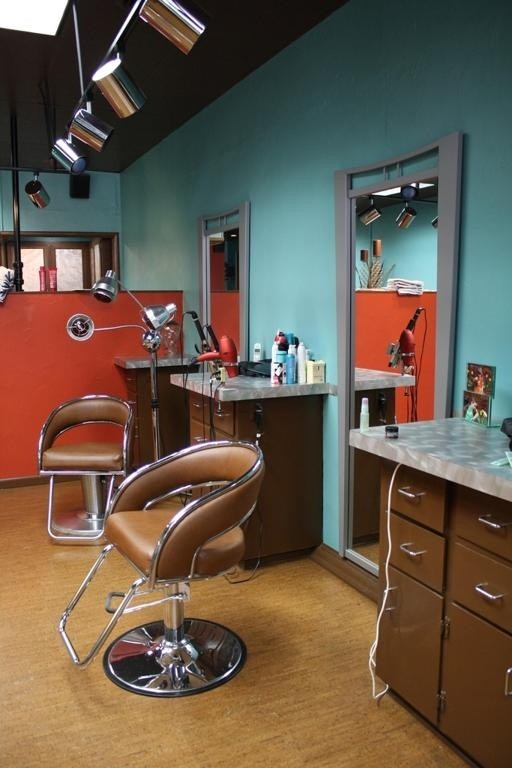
[38,394,134,541]
[58,439,265,696]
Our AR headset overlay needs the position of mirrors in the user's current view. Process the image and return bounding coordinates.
[332,128,461,577]
[198,197,253,361]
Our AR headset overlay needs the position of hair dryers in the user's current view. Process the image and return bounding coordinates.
[400,329,416,372]
[196,336,238,377]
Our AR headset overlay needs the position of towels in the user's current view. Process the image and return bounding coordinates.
[385,275,424,295]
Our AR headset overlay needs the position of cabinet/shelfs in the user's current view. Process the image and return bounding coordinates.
[188,392,325,560]
[120,365,198,469]
[438,484,512,768]
[353,388,415,541]
[371,456,445,732]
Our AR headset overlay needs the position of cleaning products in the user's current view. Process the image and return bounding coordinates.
[39,266,46,292]
[286,343,307,384]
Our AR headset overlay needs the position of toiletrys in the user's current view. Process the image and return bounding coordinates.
[39,266,57,291]
[269,330,325,386]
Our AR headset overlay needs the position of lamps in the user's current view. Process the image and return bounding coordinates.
[24,169,51,209]
[359,192,438,228]
[66,268,181,461]
[50,1,207,176]
